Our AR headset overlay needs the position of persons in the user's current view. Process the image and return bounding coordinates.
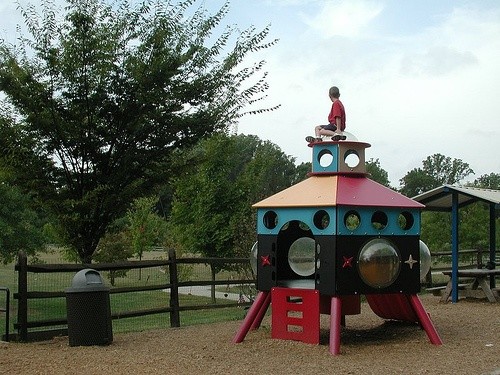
[305,87,347,141]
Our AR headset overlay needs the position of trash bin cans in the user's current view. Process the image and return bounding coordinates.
[66,268,114,348]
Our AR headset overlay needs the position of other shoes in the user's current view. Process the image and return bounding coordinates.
[305,136,322,143]
[331,135,346,141]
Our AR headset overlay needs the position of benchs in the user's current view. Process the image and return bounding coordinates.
[438,268,500,304]
